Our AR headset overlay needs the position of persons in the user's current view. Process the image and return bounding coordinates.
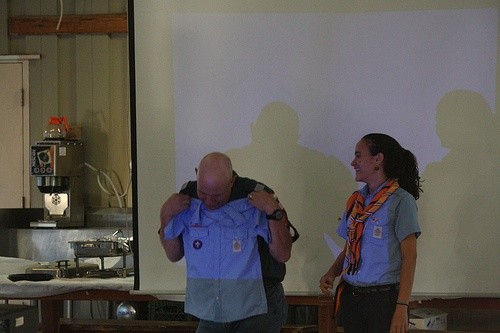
[318,133,425,333]
[157,151,300,333]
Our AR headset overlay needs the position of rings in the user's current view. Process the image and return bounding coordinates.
[248,194,254,198]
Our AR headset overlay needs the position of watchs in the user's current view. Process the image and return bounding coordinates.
[265,208,285,220]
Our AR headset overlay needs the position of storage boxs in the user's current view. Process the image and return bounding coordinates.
[408,307,448,332]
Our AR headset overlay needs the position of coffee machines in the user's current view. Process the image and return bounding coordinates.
[28,140,85,229]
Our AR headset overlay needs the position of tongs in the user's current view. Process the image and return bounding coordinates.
[111,229,124,238]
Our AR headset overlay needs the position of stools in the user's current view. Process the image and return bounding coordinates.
[0,303,38,333]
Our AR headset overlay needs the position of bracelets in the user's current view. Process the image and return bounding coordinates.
[396,301,410,307]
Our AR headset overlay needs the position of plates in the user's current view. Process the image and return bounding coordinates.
[7,273,53,282]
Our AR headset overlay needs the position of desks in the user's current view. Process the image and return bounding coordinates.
[0,256,437,333]
